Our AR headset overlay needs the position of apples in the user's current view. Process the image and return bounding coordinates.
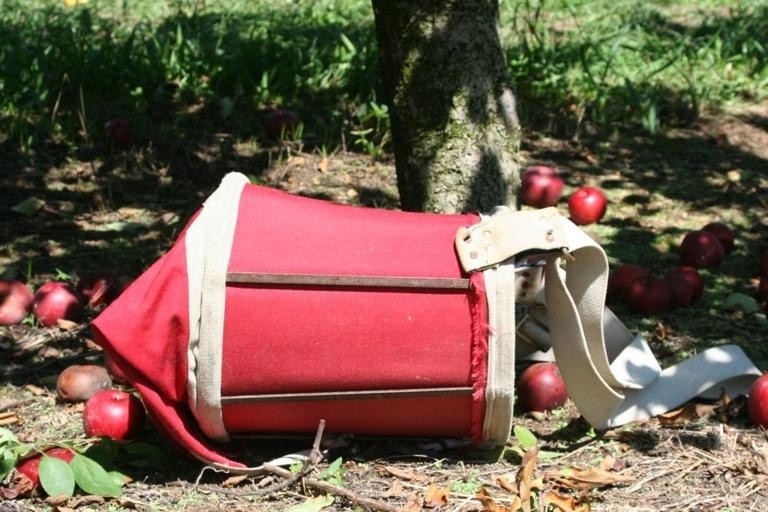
[0,273,146,497]
[269,109,299,137]
[569,187,607,224]
[518,363,567,411]
[613,223,733,314]
[747,373,768,429]
[520,165,556,182]
[520,174,564,207]
[104,118,130,144]
[758,249,768,298]
